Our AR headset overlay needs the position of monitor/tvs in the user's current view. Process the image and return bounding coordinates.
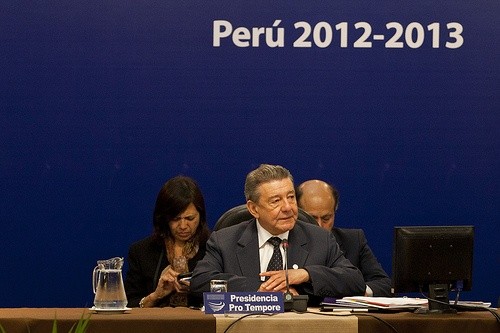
[391,225,474,313]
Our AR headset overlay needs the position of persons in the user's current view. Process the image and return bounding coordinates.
[190,164,366,308]
[125,176,212,309]
[294,180,394,298]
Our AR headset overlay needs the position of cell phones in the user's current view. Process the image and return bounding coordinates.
[178,272,193,283]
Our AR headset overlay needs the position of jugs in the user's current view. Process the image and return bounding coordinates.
[92,257,128,310]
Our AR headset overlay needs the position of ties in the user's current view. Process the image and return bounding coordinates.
[265,236,283,282]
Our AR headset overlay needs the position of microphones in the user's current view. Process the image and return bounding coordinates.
[280,238,310,313]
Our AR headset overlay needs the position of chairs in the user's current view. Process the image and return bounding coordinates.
[212,204,320,231]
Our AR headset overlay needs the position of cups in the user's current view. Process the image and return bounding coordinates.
[210,279,228,293]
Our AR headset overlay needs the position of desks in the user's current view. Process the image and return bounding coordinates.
[0,301,500,333]
